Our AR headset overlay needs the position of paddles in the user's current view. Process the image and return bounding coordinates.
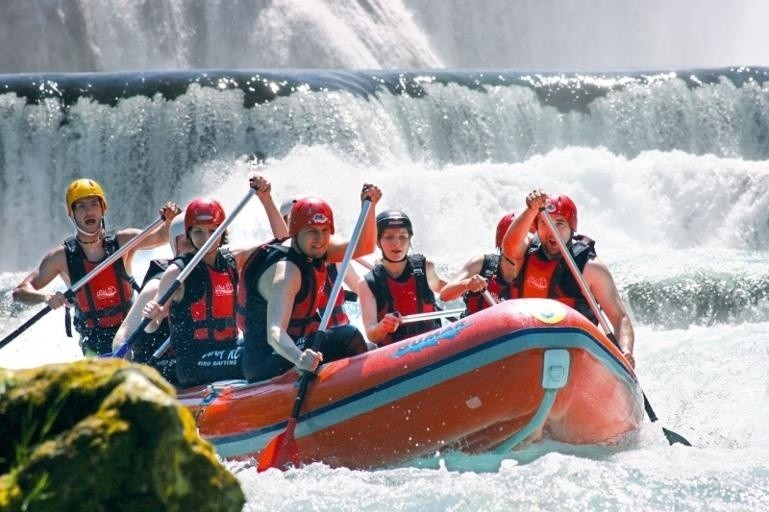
[533,205,690,445]
[257,196,373,470]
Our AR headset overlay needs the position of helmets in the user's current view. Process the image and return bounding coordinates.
[495,212,536,248]
[169,199,224,258]
[280,197,335,237]
[534,192,577,232]
[376,209,413,247]
[66,178,109,219]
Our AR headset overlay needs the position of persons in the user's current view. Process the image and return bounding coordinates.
[438,213,538,321]
[12,178,179,364]
[501,187,634,370]
[143,175,288,388]
[281,198,360,301]
[112,214,194,362]
[239,183,383,380]
[357,210,452,352]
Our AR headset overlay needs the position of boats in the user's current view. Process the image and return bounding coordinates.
[176,298,646,482]
